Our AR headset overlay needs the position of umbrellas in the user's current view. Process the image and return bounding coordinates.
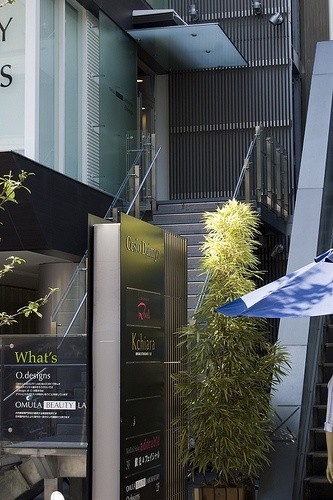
[213,244,333,318]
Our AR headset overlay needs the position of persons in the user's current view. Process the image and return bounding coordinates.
[314,366,333,499]
